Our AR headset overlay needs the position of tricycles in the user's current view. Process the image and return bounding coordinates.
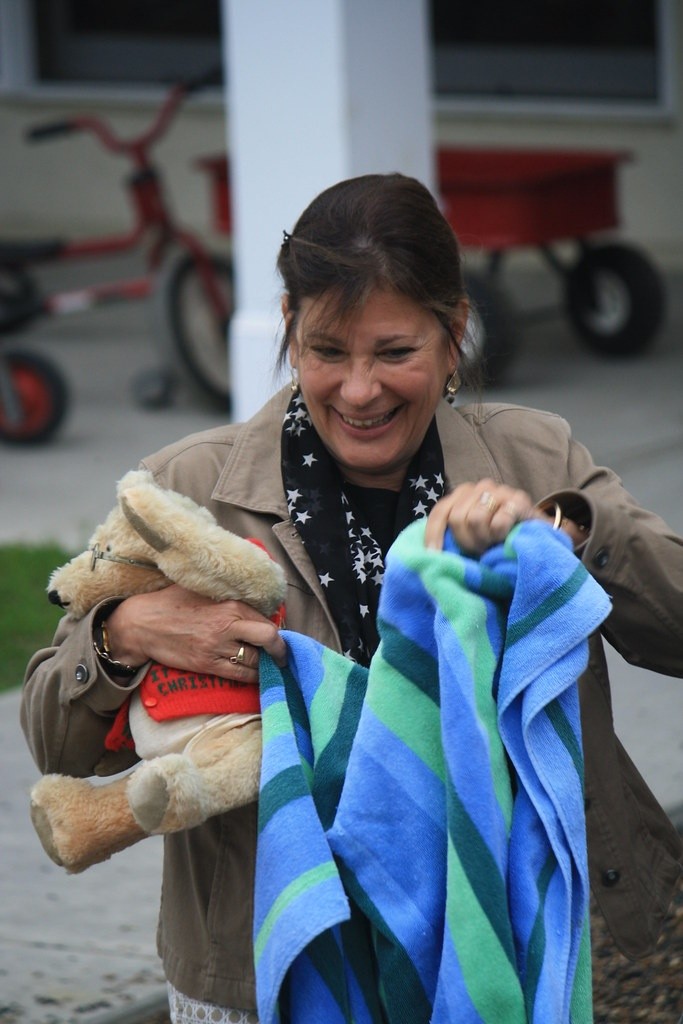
[0,65,235,445]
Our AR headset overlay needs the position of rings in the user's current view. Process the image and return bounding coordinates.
[480,494,500,510]
[230,645,246,664]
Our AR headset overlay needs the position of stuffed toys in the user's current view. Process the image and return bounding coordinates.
[30,469,287,874]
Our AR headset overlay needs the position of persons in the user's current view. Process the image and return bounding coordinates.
[19,172,683,1024]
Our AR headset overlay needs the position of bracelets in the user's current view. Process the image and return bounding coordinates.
[93,619,137,677]
[553,501,562,530]
